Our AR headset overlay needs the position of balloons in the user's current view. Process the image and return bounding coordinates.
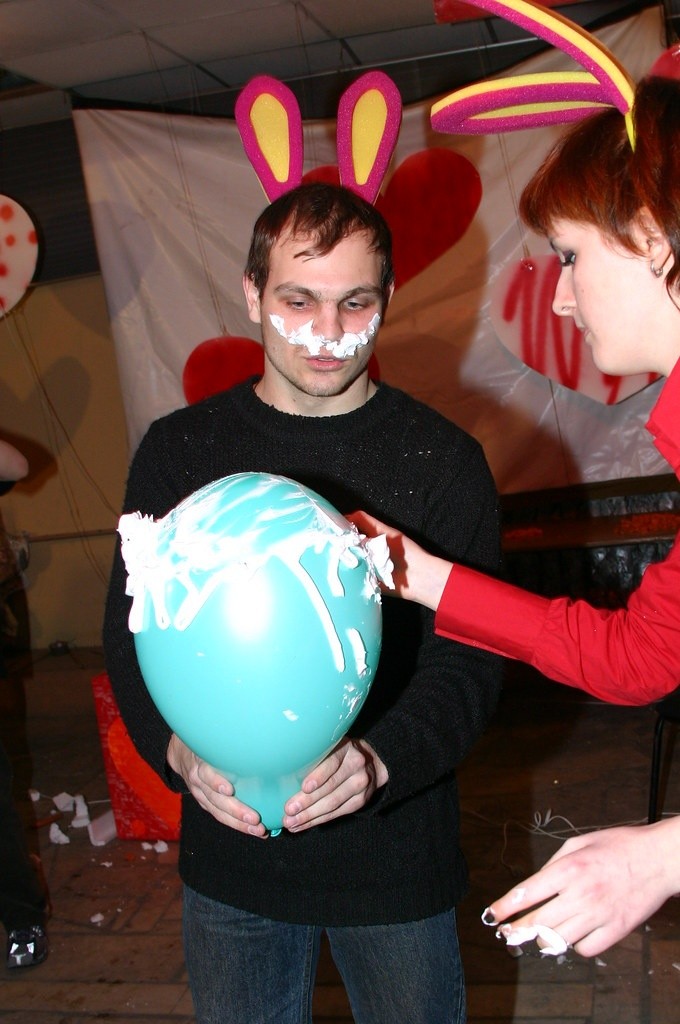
[0,194,38,319]
[115,466,384,836]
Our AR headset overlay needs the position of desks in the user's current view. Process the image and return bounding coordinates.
[502,511,680,825]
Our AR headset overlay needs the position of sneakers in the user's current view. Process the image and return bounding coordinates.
[6,926,49,967]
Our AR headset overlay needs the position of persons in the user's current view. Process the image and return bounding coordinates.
[0,521,48,981]
[344,71,680,966]
[101,180,511,1024]
